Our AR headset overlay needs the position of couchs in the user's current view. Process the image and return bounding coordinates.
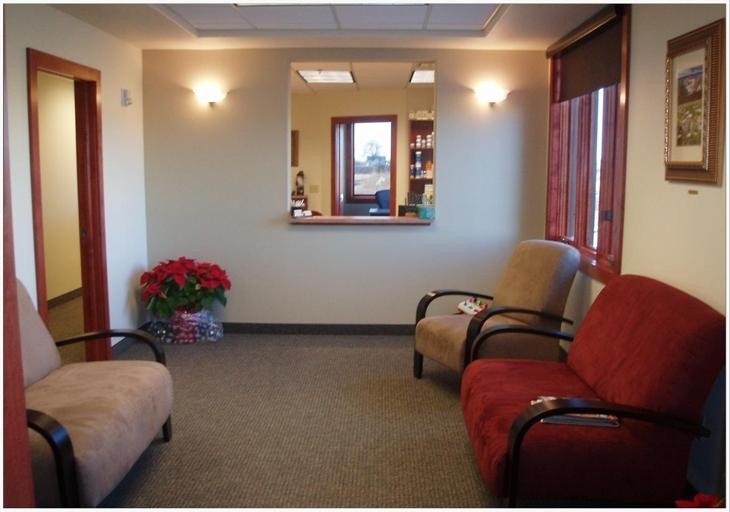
[460,275,726,508]
[14,277,173,508]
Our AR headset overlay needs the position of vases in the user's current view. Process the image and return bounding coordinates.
[172,307,197,343]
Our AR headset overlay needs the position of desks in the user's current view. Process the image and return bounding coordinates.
[368,208,389,216]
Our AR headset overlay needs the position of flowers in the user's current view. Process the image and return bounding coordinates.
[141,257,231,318]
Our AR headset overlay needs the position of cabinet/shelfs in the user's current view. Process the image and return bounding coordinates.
[408,119,433,195]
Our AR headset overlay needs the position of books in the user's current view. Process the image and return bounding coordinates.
[532,395,620,427]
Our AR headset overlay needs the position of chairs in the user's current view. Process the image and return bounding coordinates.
[413,239,582,379]
[376,189,390,210]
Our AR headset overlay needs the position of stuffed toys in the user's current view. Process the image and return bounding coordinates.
[455,296,488,316]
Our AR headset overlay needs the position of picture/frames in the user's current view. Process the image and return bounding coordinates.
[664,17,726,186]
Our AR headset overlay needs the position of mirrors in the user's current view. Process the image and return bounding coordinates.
[287,59,436,222]
